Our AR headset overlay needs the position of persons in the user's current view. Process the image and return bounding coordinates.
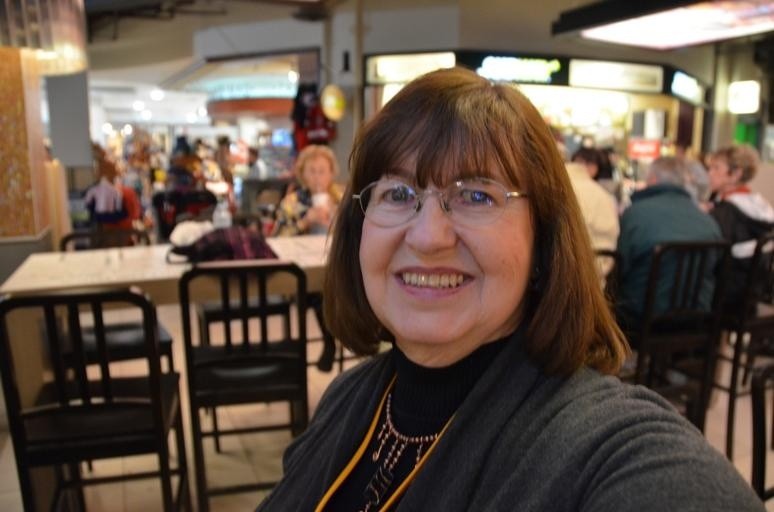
[84,133,267,222]
[277,145,347,374]
[561,140,774,340]
[250,64,766,512]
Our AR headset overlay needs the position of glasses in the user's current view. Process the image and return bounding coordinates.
[350,175,526,230]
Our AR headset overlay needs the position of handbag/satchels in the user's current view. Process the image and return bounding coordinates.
[165,215,278,266]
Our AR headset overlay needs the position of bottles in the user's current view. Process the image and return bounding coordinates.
[212,198,233,229]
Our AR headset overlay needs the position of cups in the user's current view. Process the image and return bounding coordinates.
[311,193,330,224]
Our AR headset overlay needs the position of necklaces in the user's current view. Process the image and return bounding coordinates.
[356,391,441,511]
